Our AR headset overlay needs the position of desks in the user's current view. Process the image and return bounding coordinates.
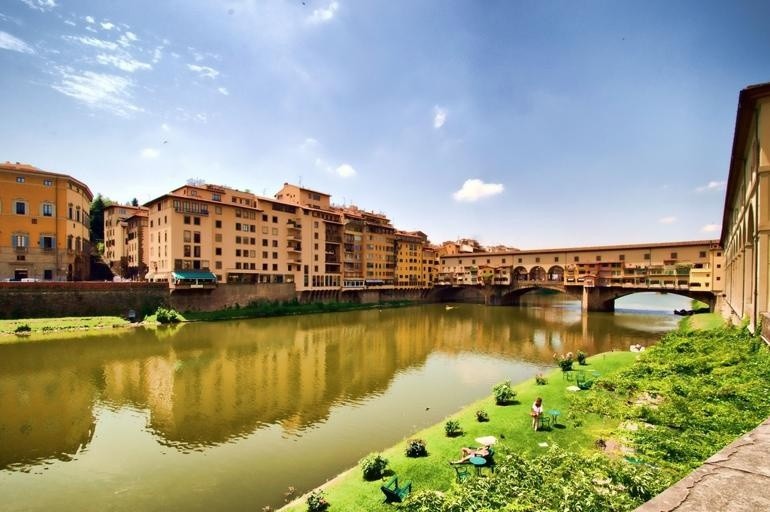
[548,409,561,424]
[470,457,487,476]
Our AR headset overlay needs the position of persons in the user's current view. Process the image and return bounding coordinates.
[531,397,543,432]
[451,444,490,464]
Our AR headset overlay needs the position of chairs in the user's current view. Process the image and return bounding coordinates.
[541,415,550,431]
[380,476,411,504]
[453,465,471,484]
[463,447,494,463]
